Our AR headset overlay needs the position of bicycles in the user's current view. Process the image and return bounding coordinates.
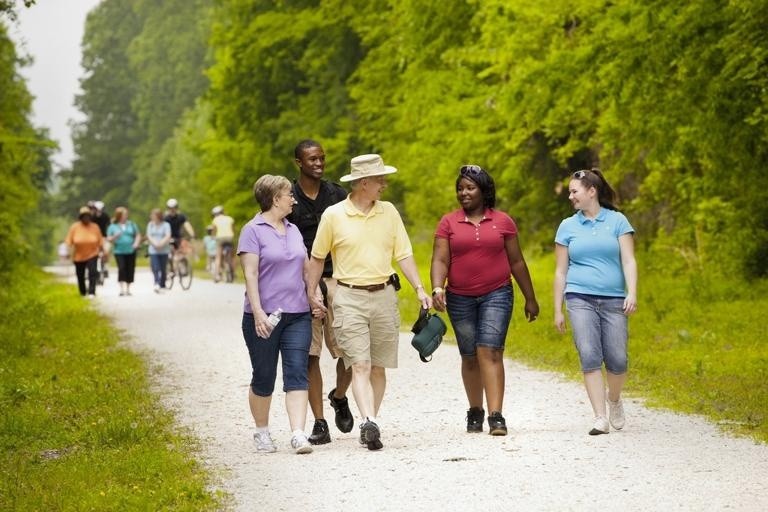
[160,235,197,291]
[217,247,237,283]
[90,256,109,287]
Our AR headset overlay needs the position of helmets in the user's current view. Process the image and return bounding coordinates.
[166,198,178,208]
[210,205,224,218]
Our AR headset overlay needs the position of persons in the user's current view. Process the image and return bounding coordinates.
[235,174,324,454]
[552,167,637,435]
[430,166,539,435]
[290,139,353,446]
[306,154,433,449]
[55,199,234,299]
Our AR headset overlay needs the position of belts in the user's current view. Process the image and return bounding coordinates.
[337,279,392,292]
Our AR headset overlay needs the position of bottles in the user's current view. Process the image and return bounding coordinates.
[261,308,284,339]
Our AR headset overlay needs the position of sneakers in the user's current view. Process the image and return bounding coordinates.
[290,433,314,454]
[605,388,625,430]
[488,411,507,435]
[358,416,383,450]
[328,388,353,433]
[588,414,610,435]
[308,419,331,445]
[254,431,277,454]
[464,406,484,433]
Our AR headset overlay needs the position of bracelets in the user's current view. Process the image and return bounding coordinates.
[416,285,424,289]
[432,287,445,295]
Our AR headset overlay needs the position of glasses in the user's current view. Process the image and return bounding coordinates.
[573,170,586,180]
[281,192,293,199]
[461,165,484,177]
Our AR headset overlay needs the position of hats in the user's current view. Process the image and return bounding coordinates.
[339,153,397,183]
[78,206,92,219]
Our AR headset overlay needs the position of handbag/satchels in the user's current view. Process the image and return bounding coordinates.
[410,304,447,362]
